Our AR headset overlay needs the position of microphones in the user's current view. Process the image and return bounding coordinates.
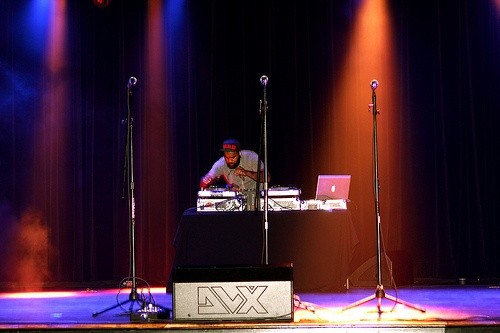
[370,79,379,89]
[127,77,137,88]
[260,75,269,85]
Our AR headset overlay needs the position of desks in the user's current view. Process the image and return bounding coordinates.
[167,208,350,293]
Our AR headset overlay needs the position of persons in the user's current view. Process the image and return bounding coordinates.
[200,140,270,190]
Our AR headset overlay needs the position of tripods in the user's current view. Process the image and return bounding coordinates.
[341,87,427,316]
[92,83,176,318]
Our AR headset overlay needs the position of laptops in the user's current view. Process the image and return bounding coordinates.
[316,175,351,199]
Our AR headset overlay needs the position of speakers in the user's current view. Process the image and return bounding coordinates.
[173,262,293,321]
[350,250,412,287]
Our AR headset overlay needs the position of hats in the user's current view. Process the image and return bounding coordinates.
[223,140,239,152]
